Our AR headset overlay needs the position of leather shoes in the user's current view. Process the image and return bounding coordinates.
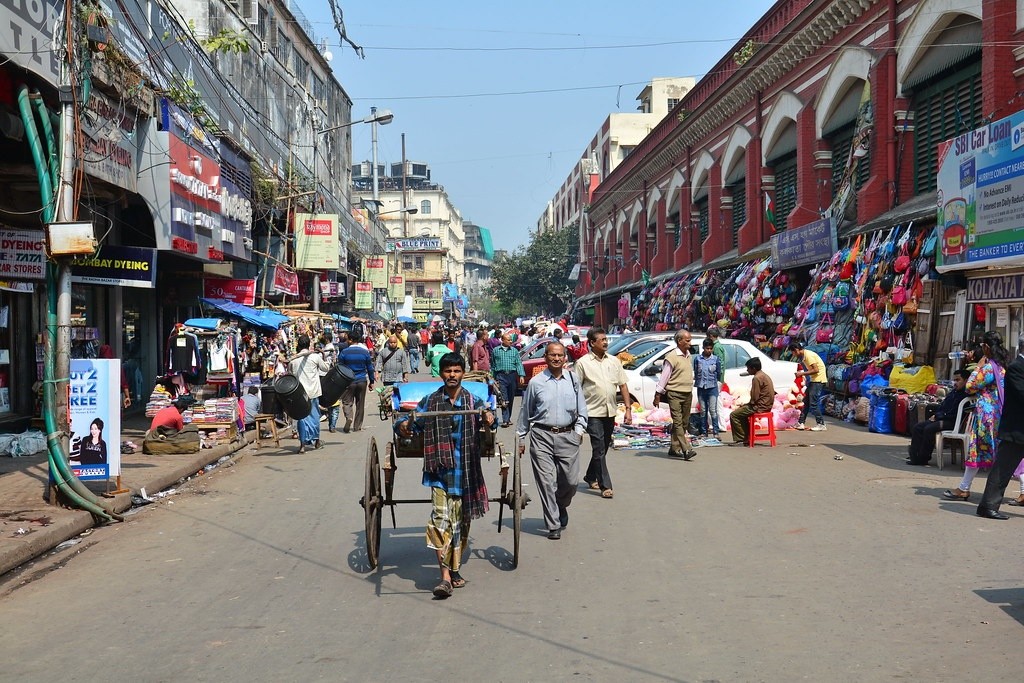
[976,506,1010,520]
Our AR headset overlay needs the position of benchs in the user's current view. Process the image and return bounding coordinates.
[393,382,496,457]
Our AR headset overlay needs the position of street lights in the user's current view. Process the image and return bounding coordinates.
[311,107,394,311]
[373,199,418,314]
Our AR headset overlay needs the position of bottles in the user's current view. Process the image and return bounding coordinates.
[948,351,967,359]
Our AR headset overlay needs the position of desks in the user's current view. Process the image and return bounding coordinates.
[190,417,239,444]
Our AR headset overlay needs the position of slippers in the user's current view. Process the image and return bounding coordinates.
[450,571,465,587]
[601,488,613,497]
[583,476,600,489]
[315,440,325,449]
[299,450,305,455]
[944,489,970,501]
[1009,499,1024,506]
[433,579,453,597]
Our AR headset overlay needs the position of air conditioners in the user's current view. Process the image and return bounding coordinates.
[314,99,318,107]
[261,42,268,53]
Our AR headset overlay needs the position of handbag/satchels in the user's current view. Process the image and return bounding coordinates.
[779,221,938,435]
[628,257,797,356]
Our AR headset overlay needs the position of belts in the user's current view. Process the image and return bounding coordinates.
[535,422,572,434]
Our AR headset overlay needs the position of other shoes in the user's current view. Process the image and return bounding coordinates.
[509,420,513,425]
[683,450,697,460]
[906,457,928,466]
[668,449,685,459]
[729,439,748,446]
[329,429,336,433]
[501,423,509,427]
[547,528,561,539]
[700,434,707,438]
[344,419,353,433]
[715,434,722,442]
[559,507,569,527]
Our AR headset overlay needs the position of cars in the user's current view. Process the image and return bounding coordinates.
[512,323,807,431]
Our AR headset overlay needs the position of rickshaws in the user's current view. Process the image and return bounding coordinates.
[358,378,531,568]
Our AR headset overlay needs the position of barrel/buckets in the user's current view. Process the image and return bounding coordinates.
[319,363,356,407]
[274,374,312,420]
[261,387,284,421]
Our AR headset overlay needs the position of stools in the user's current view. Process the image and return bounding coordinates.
[255,414,279,450]
[750,412,776,448]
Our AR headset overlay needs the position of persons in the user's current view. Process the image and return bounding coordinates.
[69,418,107,464]
[392,352,499,598]
[98,316,1023,549]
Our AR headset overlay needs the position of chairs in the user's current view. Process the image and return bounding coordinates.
[936,397,977,471]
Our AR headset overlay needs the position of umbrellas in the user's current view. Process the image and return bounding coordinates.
[428,315,445,321]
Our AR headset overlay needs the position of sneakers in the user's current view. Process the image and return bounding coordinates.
[810,423,828,431]
[793,422,804,429]
